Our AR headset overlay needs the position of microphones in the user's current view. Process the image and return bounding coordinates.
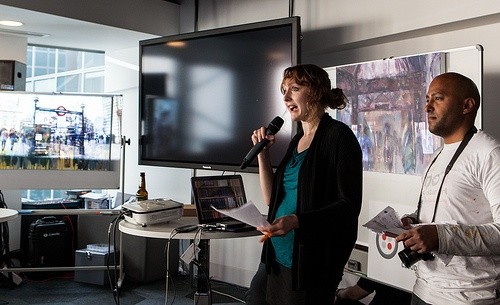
[242,116,284,168]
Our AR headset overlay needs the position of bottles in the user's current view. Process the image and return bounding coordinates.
[135,172,148,201]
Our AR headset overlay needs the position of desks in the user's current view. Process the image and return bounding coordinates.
[119,215,265,305]
[0,208,125,295]
[0,208,18,292]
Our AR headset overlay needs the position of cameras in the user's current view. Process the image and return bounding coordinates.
[398,247,435,267]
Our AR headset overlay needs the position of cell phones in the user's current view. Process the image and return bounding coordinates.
[176,224,197,232]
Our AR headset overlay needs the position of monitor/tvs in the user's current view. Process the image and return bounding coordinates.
[139,17,304,174]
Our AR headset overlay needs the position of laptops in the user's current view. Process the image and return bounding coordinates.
[190,176,257,233]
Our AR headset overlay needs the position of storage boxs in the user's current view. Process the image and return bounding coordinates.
[367,229,416,294]
[183,205,196,216]
[74,249,118,285]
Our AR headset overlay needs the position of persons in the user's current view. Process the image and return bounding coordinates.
[395,72,500,305]
[244,63,362,305]
[335,276,412,305]
[0,128,35,151]
